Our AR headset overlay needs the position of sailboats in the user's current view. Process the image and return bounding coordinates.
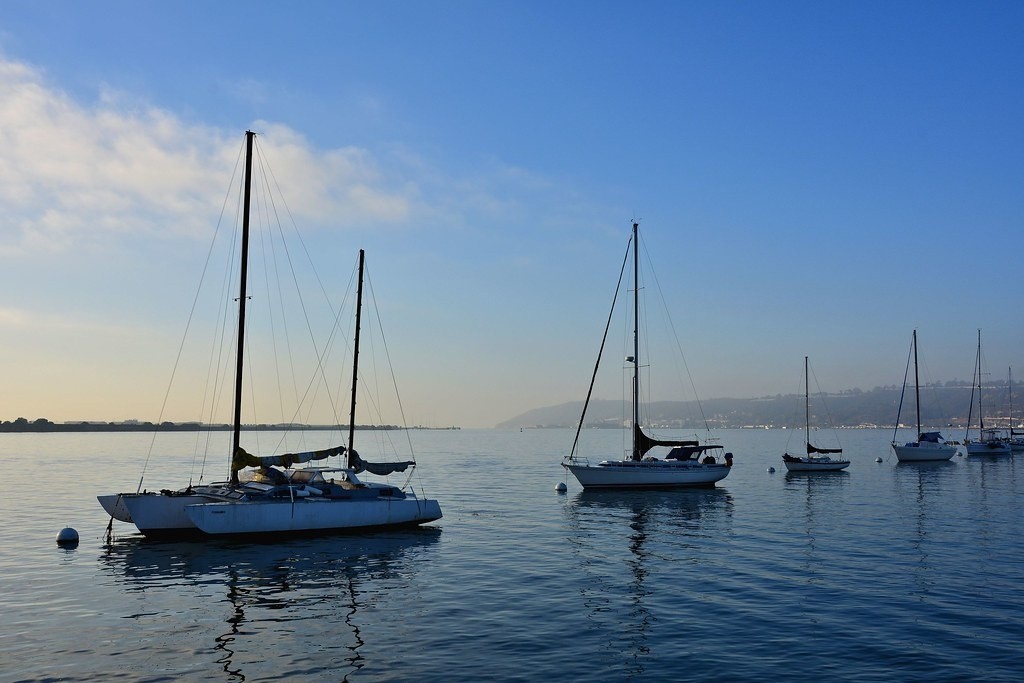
[559,219,733,492]
[889,329,957,462]
[961,328,1024,456]
[780,356,852,472]
[96,130,444,545]
